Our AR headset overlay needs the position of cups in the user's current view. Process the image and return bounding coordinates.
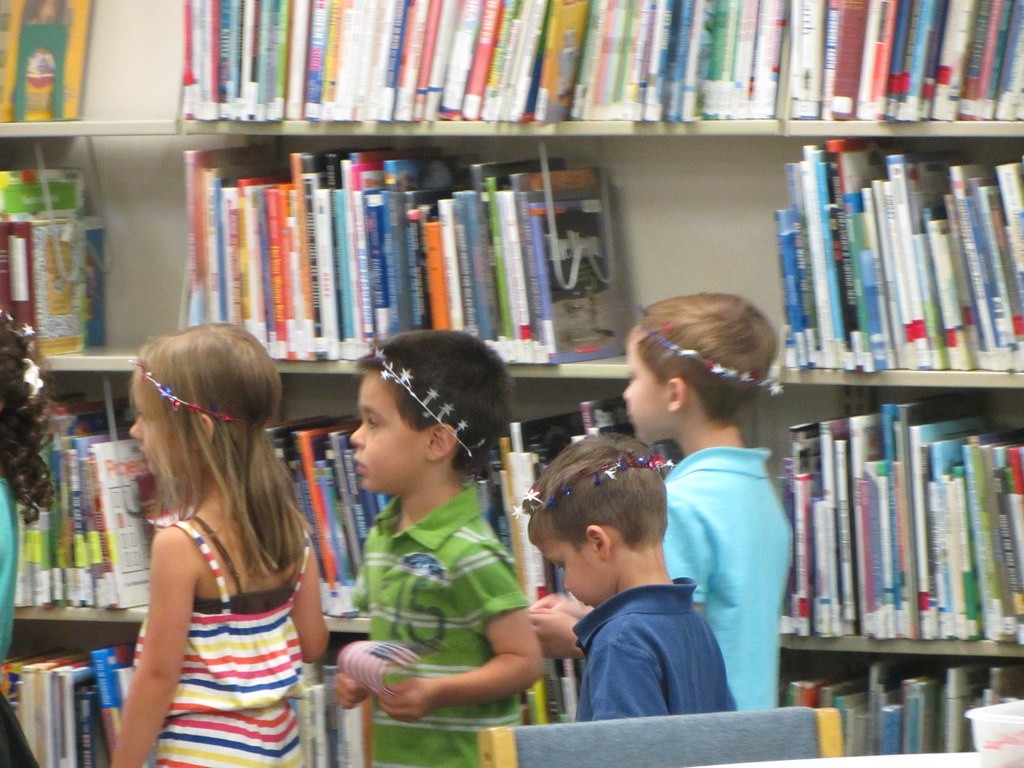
[964,700,1024,768]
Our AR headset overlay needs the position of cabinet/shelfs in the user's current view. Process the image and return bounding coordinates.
[0,0,1024,668]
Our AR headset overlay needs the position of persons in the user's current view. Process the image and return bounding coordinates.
[108,324,329,768]
[526,292,794,713]
[334,328,544,768]
[512,433,736,722]
[0,315,54,768]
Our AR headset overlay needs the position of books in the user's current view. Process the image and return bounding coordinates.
[773,138,1024,373]
[774,663,1024,758]
[780,396,1024,644]
[0,1,1024,123]
[0,395,622,765]
[0,143,625,366]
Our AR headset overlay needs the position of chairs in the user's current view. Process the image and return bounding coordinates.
[478,705,842,768]
